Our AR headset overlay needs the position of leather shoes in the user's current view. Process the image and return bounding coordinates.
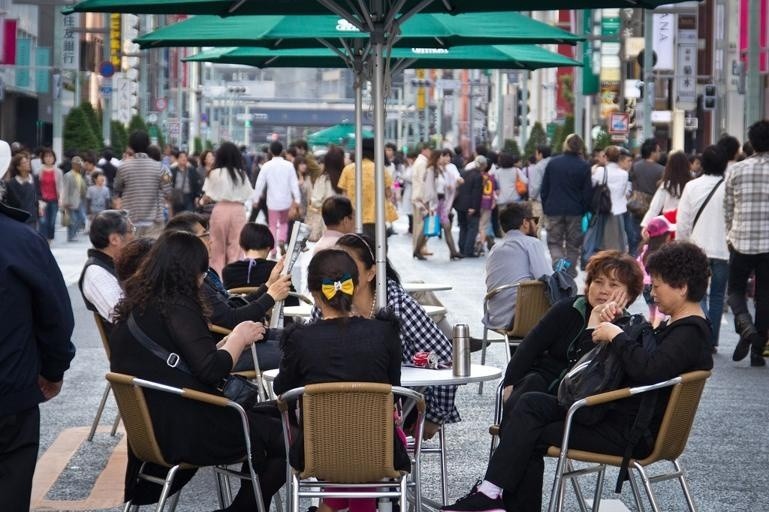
[732,325,757,360]
[752,358,765,365]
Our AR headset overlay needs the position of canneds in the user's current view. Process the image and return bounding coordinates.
[410,350,439,367]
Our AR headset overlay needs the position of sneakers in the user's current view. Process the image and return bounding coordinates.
[441,483,507,511]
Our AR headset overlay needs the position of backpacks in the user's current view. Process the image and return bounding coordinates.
[557,313,655,495]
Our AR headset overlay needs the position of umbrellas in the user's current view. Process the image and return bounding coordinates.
[129,12,588,235]
[179,42,586,100]
[59,0,696,314]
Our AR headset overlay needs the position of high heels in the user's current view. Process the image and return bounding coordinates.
[413,252,427,261]
[451,252,467,260]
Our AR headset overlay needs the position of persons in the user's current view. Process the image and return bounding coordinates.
[1,131,769,512]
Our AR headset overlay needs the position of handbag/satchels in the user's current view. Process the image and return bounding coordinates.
[288,205,304,222]
[627,189,654,217]
[62,208,71,226]
[516,179,528,195]
[589,184,610,214]
[171,190,185,215]
[303,205,324,241]
[222,374,258,410]
[423,211,440,238]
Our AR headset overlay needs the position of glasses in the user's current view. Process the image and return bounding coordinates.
[196,232,211,239]
[116,226,136,237]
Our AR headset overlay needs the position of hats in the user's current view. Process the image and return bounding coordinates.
[0,140,12,180]
[647,215,677,237]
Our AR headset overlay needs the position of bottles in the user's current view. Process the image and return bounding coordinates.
[452,323,471,376]
